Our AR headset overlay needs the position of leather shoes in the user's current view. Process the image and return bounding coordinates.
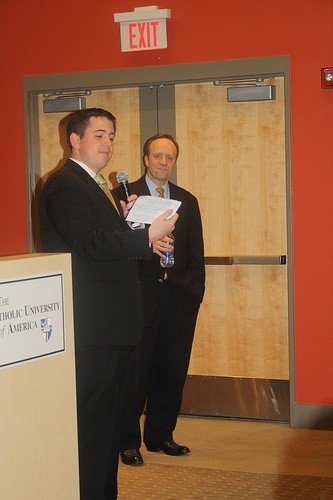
[146,441,190,455]
[120,448,144,466]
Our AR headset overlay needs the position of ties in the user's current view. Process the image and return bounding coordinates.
[156,187,165,199]
[93,174,121,216]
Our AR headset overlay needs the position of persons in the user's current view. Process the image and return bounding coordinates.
[34,107,180,500]
[111,134,207,466]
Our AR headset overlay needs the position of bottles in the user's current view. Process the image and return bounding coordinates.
[159,232,175,268]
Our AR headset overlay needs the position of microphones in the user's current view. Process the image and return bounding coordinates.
[116,172,141,228]
